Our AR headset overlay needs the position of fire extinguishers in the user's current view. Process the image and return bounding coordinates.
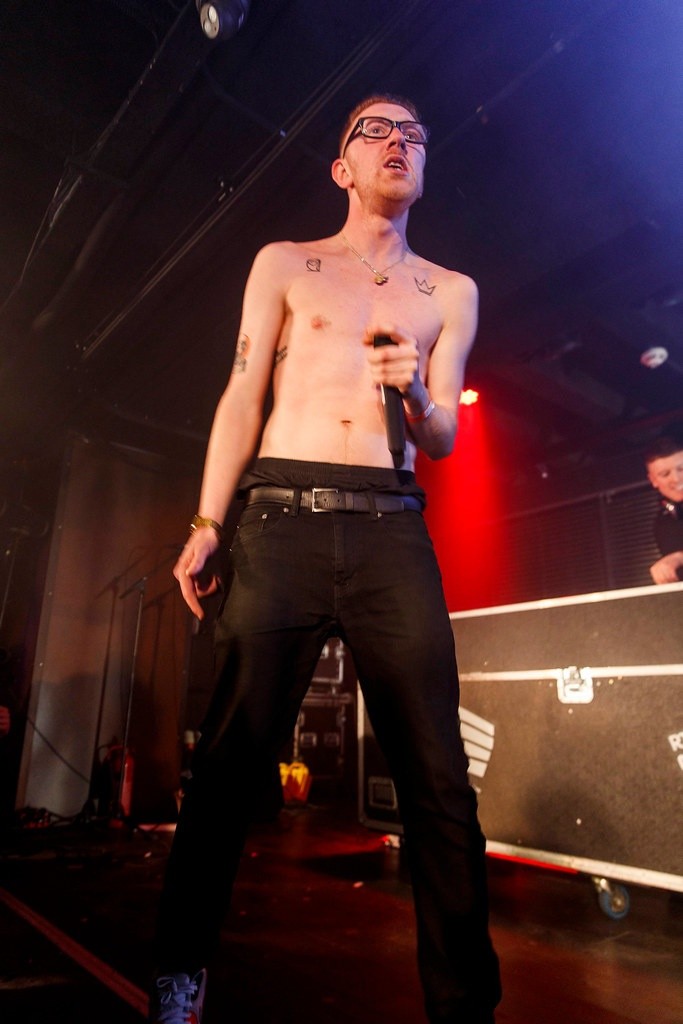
[96,736,137,828]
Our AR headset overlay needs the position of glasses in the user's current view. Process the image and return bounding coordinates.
[342,116,430,156]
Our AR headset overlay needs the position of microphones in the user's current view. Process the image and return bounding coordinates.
[371,334,410,469]
[163,542,186,549]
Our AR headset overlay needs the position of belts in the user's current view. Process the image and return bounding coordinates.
[246,485,424,514]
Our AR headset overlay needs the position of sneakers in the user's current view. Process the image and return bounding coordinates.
[156,967,206,1024]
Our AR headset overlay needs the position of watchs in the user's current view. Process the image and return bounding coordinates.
[190,514,224,540]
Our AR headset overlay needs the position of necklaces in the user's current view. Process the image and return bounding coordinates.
[339,232,409,285]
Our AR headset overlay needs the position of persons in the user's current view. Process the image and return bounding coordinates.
[151,97,502,1024]
[644,436,683,585]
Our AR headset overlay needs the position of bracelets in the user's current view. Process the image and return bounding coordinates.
[404,399,436,423]
